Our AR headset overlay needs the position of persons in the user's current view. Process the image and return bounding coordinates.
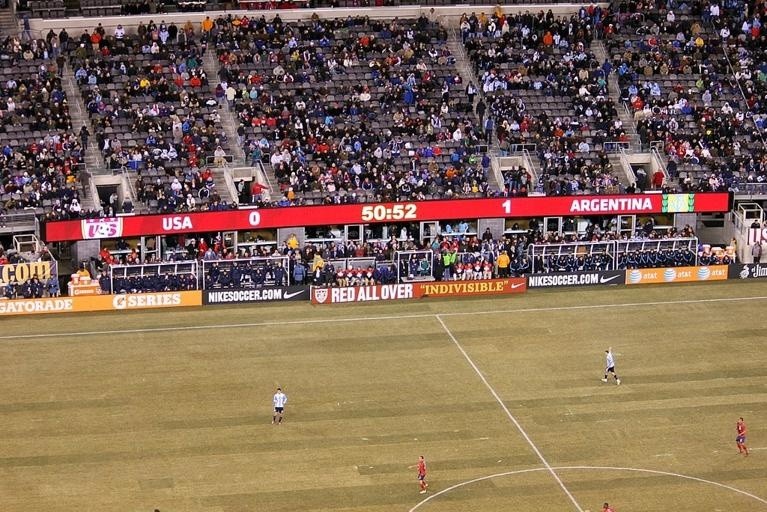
[598,346,621,387]
[416,454,429,494]
[271,387,287,424]
[603,502,614,512]
[734,417,750,458]
[0,0,765,300]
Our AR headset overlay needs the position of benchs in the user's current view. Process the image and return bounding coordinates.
[0,279,61,299]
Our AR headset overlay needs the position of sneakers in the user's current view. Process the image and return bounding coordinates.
[420,484,428,493]
[601,379,607,383]
[617,379,621,386]
[272,421,282,425]
[740,451,749,457]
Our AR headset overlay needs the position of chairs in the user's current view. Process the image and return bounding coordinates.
[0,0,767,221]
[67,226,736,297]
